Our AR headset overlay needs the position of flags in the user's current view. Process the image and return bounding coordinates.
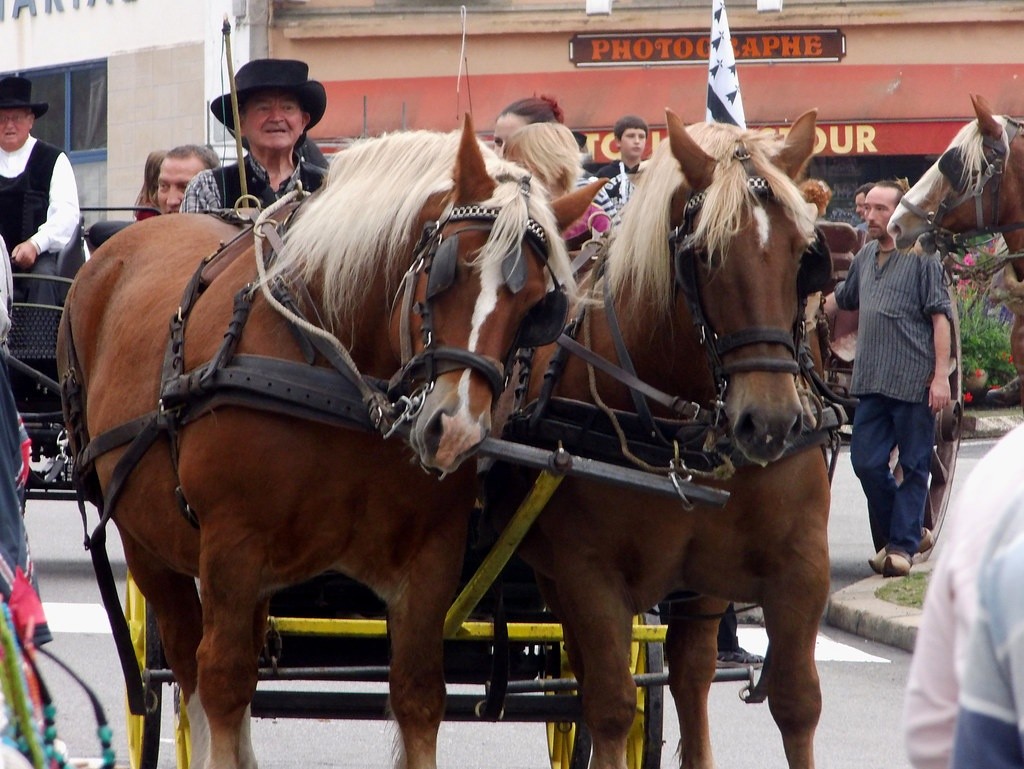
[706,0,747,129]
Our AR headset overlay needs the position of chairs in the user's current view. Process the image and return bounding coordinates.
[817,220,872,271]
[56,223,84,306]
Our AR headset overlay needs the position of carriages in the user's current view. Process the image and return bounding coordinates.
[5,103,964,769]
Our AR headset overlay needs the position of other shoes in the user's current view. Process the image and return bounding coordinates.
[882,554,910,576]
[715,648,765,667]
[868,528,936,573]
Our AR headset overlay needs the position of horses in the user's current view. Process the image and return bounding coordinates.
[52,107,835,768]
[887,89,1023,385]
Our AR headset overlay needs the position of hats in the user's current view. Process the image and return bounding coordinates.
[210,59,327,131]
[0,77,49,119]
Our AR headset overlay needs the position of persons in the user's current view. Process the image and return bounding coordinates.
[492,96,648,253]
[795,180,951,578]
[179,59,329,213]
[0,77,80,306]
[133,144,222,220]
